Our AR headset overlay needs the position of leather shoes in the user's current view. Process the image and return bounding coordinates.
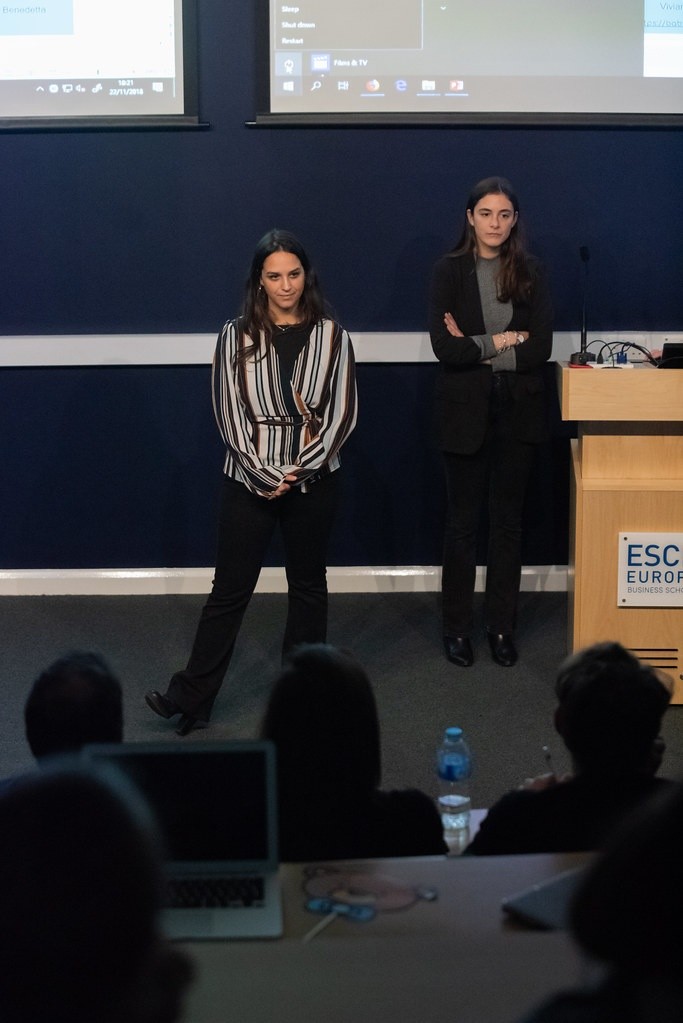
[442,630,474,667]
[484,629,519,668]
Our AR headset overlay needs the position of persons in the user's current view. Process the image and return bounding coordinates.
[429,177,553,667]
[146,231,359,733]
[1,640,682,1022]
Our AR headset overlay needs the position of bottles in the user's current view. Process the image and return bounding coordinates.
[436,727,472,831]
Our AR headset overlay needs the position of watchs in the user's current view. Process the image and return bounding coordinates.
[513,331,524,346]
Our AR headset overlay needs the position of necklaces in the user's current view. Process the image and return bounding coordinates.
[273,319,298,331]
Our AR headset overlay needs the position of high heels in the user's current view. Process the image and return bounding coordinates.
[144,689,195,735]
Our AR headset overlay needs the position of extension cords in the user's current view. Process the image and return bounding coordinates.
[585,360,634,369]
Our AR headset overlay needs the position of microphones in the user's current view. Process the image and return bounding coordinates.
[570,246,596,366]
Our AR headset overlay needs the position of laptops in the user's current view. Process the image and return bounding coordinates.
[78,739,282,944]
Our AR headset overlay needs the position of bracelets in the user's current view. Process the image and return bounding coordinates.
[498,331,510,354]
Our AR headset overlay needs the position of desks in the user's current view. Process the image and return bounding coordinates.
[169,850,609,1022]
